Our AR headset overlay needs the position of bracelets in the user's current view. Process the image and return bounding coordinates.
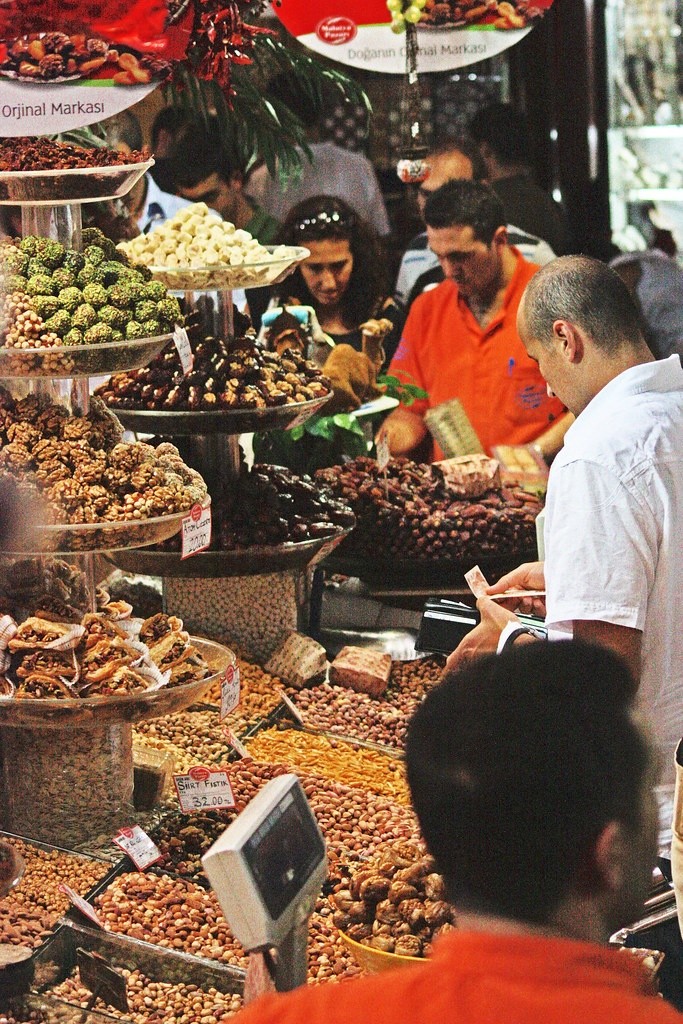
[531,443,542,458]
[497,622,525,655]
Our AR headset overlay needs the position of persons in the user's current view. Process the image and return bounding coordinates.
[247,195,405,475]
[223,637,682,1024]
[99,110,247,313]
[322,178,577,462]
[395,135,556,299]
[429,255,682,889]
[245,68,391,237]
[466,104,569,258]
[608,251,683,370]
[147,105,283,246]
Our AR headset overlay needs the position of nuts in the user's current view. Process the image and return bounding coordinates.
[0,656,454,1024]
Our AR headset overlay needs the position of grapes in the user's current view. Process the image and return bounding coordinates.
[385,0,426,34]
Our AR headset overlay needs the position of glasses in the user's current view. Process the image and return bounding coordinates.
[291,208,358,233]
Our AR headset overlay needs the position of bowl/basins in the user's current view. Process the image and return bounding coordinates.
[334,931,434,978]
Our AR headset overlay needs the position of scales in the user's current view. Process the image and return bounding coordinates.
[199,774,332,1010]
